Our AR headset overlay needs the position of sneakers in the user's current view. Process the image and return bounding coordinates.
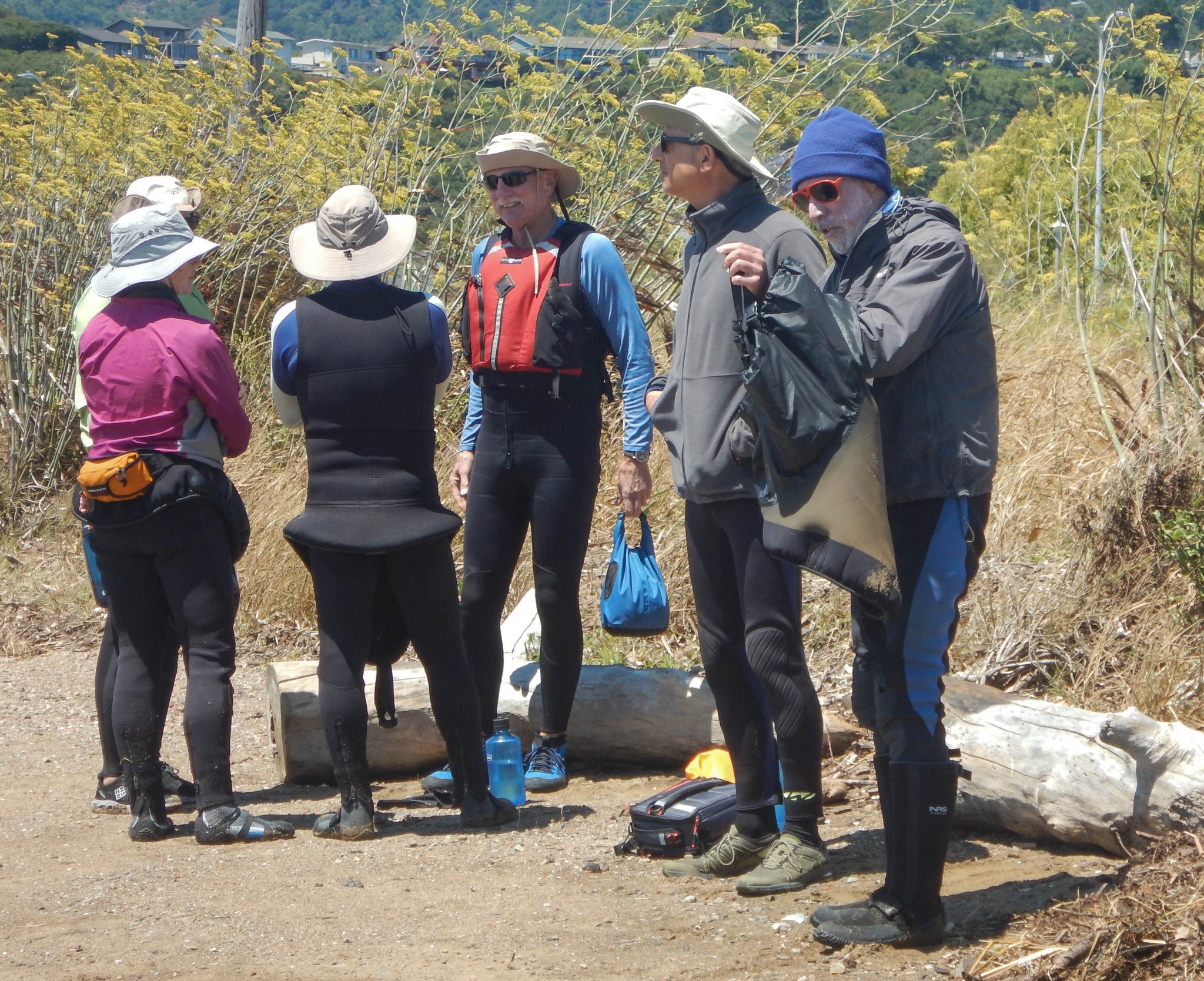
[523,730,568,794]
[736,833,835,895]
[91,774,182,813]
[159,760,197,803]
[421,745,489,791]
[662,823,782,879]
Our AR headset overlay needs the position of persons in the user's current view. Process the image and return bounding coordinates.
[69,173,295,845]
[719,109,1001,949]
[271,183,518,840]
[633,86,838,897]
[420,131,656,795]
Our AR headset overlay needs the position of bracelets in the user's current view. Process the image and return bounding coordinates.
[624,451,651,463]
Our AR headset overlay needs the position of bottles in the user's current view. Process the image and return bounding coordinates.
[81,522,109,607]
[486,713,527,807]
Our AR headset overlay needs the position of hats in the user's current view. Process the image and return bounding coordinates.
[107,176,202,238]
[790,106,894,199]
[476,132,581,201]
[634,86,780,182]
[91,202,221,298]
[288,185,417,281]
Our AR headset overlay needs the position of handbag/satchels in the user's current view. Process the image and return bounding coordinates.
[77,451,182,503]
[613,776,736,859]
[600,508,670,637]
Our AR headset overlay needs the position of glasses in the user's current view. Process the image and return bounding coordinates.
[183,213,199,229]
[791,175,845,214]
[659,130,721,160]
[481,168,546,190]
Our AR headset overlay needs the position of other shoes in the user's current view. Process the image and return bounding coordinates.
[461,791,519,830]
[312,801,375,840]
[194,803,295,845]
[812,891,946,947]
[809,886,903,929]
[129,804,175,841]
